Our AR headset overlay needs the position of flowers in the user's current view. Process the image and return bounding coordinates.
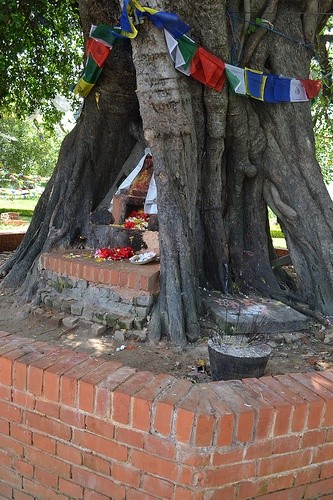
[82,245,135,263]
[124,209,151,231]
